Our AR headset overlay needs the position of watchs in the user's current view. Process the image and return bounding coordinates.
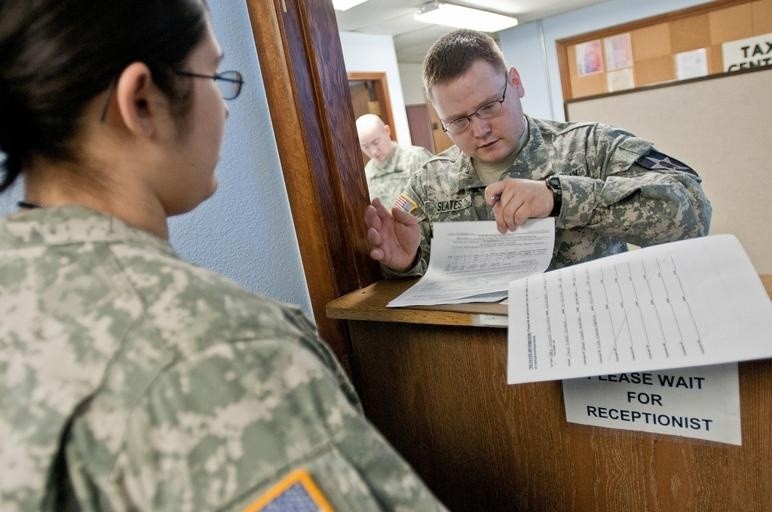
[546,175,562,217]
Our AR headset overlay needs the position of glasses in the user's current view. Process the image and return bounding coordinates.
[439,69,508,136]
[98,68,244,124]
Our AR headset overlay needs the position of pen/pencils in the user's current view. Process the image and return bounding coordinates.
[490,196,501,200]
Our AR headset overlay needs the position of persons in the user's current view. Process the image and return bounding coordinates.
[0,0,449,512]
[366,28,713,276]
[355,114,434,214]
